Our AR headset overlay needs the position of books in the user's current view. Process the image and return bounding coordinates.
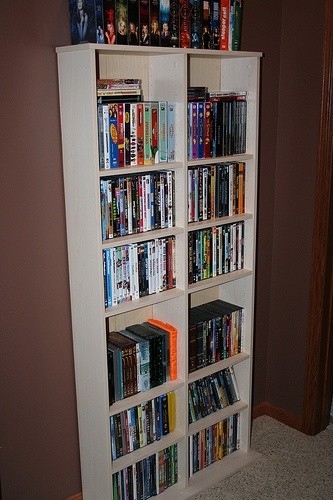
[68,0,243,51]
[94,77,246,500]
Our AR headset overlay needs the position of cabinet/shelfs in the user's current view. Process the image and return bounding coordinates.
[55,42,260,499]
